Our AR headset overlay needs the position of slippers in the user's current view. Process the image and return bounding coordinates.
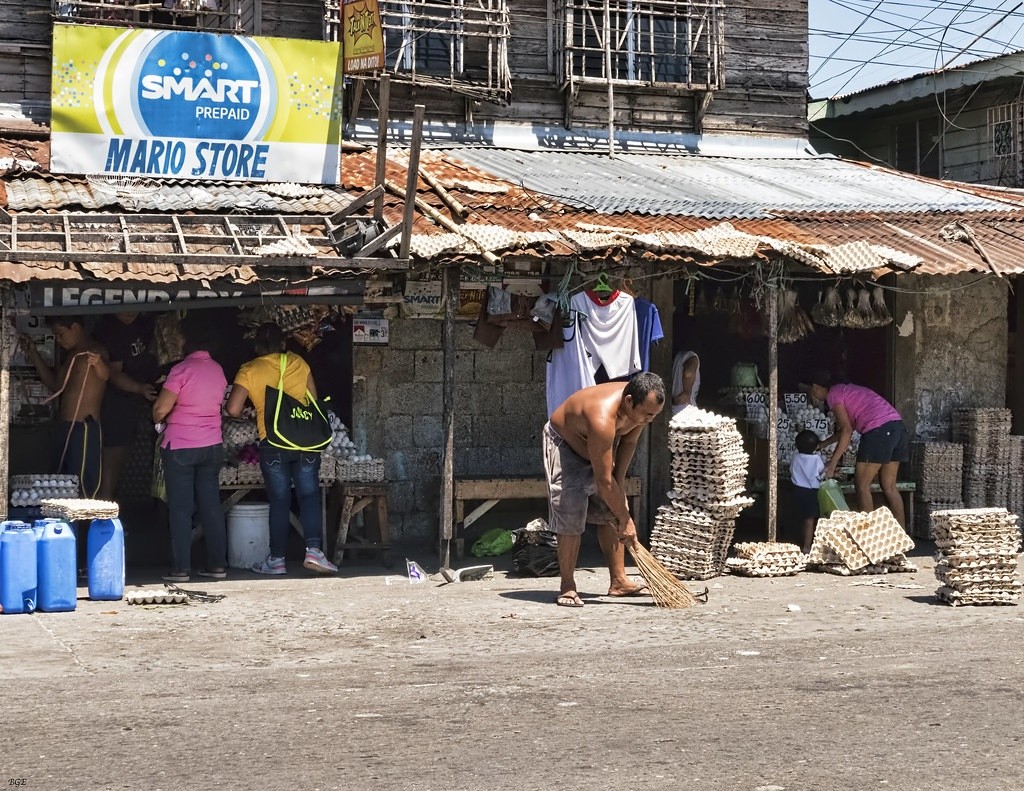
[608,584,653,597]
[555,593,584,606]
[161,570,189,581]
[196,568,226,578]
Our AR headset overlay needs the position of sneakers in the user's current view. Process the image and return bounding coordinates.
[303,547,338,574]
[251,554,287,574]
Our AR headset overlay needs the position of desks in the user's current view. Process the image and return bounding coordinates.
[836,482,917,538]
[456,477,643,560]
[190,481,392,566]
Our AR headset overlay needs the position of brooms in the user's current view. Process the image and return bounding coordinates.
[589,495,698,611]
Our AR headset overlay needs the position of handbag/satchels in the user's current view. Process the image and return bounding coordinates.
[817,475,851,519]
[151,432,168,504]
[264,352,332,452]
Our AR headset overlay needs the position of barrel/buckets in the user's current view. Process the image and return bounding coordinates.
[88,518,126,600]
[227,502,271,568]
[0,520,37,613]
[33,520,77,611]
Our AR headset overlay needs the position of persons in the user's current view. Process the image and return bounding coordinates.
[789,430,846,553]
[672,315,701,416]
[18,314,109,498]
[809,366,909,530]
[543,371,665,608]
[153,317,228,580]
[93,310,158,501]
[226,326,339,575]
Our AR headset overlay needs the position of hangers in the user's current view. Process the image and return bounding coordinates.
[624,278,637,297]
[594,272,614,302]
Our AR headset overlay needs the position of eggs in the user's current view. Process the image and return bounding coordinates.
[12,479,75,499]
[325,411,372,462]
[745,403,852,464]
